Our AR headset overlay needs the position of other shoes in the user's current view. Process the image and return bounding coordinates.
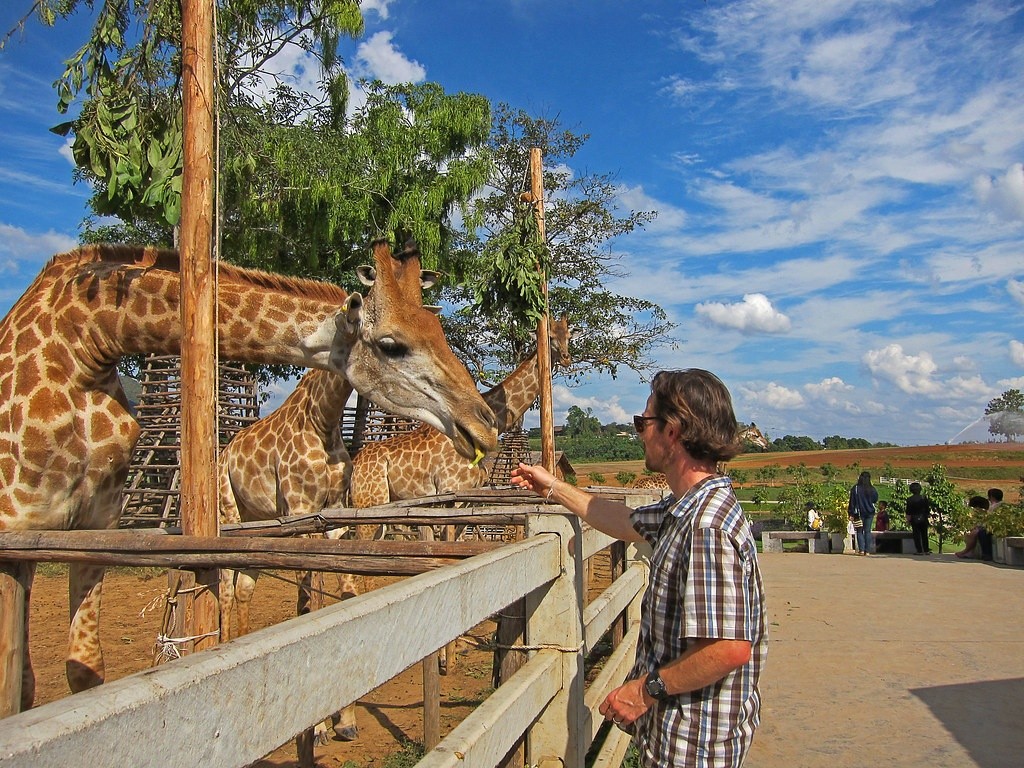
[865,552,870,556]
[955,550,974,559]
[925,552,930,555]
[914,552,923,555]
[856,551,865,555]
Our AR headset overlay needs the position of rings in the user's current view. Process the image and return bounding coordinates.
[613,717,620,724]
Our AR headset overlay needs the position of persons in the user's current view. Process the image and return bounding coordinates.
[803,501,823,531]
[907,482,932,555]
[873,501,890,532]
[510,367,769,768]
[955,488,1004,562]
[848,471,878,556]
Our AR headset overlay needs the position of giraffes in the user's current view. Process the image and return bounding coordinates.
[632,422,769,490]
[336,311,583,601]
[0,236,498,746]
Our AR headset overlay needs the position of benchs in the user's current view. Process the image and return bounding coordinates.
[967,536,1024,565]
[761,530,829,554]
[848,530,924,555]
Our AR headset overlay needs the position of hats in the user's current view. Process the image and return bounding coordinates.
[804,502,814,506]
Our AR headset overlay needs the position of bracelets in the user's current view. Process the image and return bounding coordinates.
[546,477,558,502]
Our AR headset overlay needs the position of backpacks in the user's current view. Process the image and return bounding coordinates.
[809,511,821,529]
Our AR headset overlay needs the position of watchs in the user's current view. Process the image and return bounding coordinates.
[645,670,669,702]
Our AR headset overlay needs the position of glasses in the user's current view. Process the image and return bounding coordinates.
[633,415,684,433]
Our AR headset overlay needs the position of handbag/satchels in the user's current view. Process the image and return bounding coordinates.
[852,516,863,531]
